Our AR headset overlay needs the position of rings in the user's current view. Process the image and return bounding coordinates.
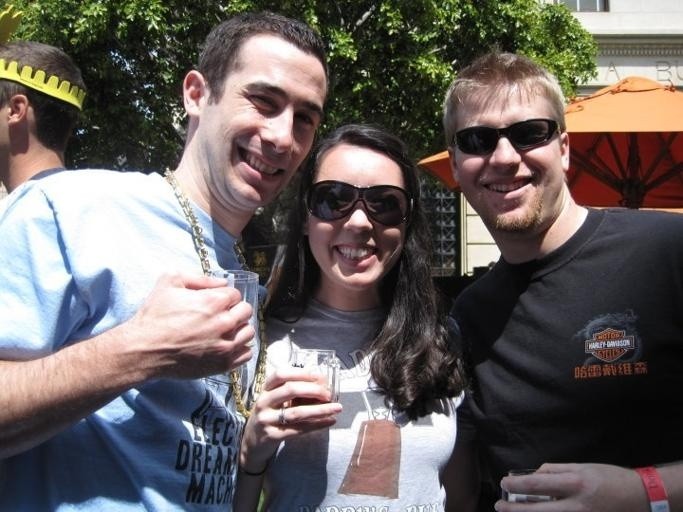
[279,408,288,426]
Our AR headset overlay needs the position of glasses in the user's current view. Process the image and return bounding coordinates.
[298,180,417,230]
[444,116,563,156]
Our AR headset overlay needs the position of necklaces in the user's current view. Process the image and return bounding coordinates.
[164,166,266,419]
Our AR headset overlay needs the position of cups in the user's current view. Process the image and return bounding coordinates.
[286,347,343,406]
[204,268,260,350]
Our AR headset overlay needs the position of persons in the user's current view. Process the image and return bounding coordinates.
[0,10,329,512]
[442,52,682,511]
[0,40,86,195]
[236,124,488,512]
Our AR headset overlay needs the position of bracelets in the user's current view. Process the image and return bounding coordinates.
[635,465,671,512]
[239,461,270,476]
